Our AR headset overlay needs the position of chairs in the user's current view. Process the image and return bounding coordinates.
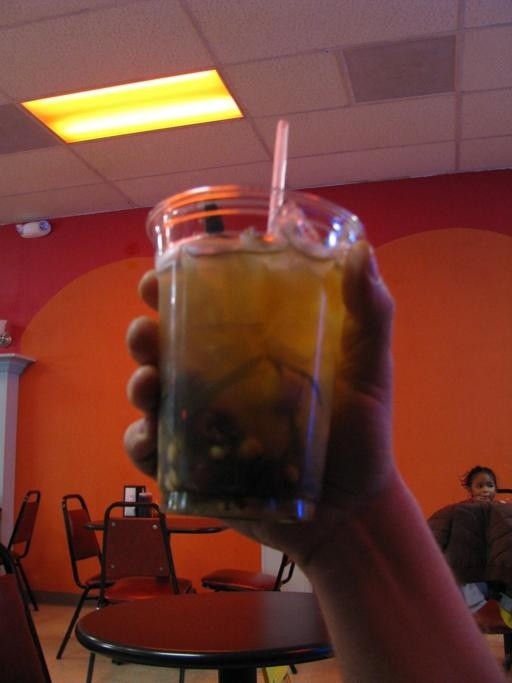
[202,552,301,682]
[86,501,195,682]
[0,488,41,613]
[56,493,158,662]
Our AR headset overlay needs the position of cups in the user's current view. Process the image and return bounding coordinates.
[138,493,151,517]
[146,184,366,526]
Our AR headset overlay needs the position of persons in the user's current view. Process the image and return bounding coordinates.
[458,467,507,504]
[124,240,512,683]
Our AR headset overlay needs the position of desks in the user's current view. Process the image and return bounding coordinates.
[78,587,334,681]
[86,514,232,537]
